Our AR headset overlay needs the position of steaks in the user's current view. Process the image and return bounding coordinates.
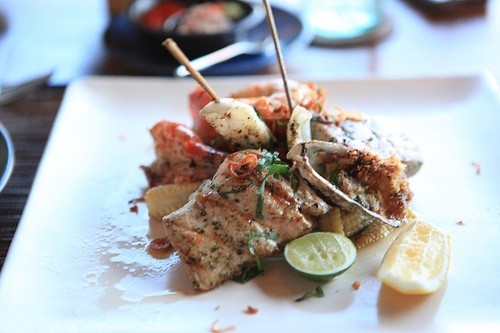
[162,148,331,291]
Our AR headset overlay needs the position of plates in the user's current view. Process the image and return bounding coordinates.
[2,77,498,333]
[104,3,315,76]
[301,4,393,47]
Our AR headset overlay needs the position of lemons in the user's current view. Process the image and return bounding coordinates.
[284,231,357,281]
[377,216,445,295]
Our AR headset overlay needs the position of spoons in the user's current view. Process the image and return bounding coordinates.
[175,33,275,78]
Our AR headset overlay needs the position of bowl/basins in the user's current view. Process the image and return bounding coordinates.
[127,0,268,56]
[0,124,16,192]
